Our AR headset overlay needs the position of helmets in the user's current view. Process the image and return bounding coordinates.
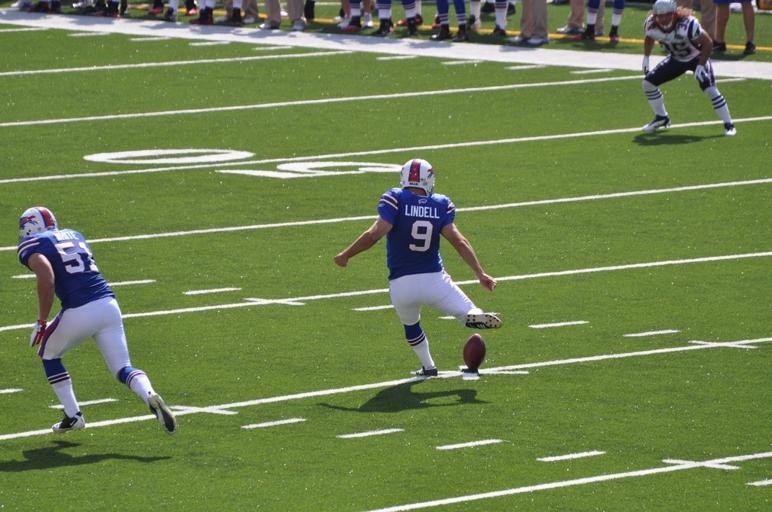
[653,0,676,14]
[400,158,434,195]
[19,207,56,237]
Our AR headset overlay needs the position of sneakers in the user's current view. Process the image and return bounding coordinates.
[724,122,736,136]
[410,366,437,375]
[642,113,670,132]
[72,4,480,43]
[148,393,176,434]
[712,40,725,51]
[744,42,756,53]
[52,410,85,432]
[466,312,502,329]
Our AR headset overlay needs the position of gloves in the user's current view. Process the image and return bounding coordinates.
[694,64,709,83]
[642,56,649,74]
[31,319,46,347]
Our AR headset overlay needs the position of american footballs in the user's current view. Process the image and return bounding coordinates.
[464,333,485,371]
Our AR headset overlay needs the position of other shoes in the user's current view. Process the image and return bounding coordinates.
[575,31,595,41]
[609,33,620,44]
[526,36,549,46]
[487,24,506,37]
[504,35,530,43]
[595,25,604,36]
[556,23,585,34]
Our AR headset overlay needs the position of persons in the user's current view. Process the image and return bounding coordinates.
[333,158,504,376]
[17,207,176,436]
[642,0,737,137]
[0,0,771,56]
